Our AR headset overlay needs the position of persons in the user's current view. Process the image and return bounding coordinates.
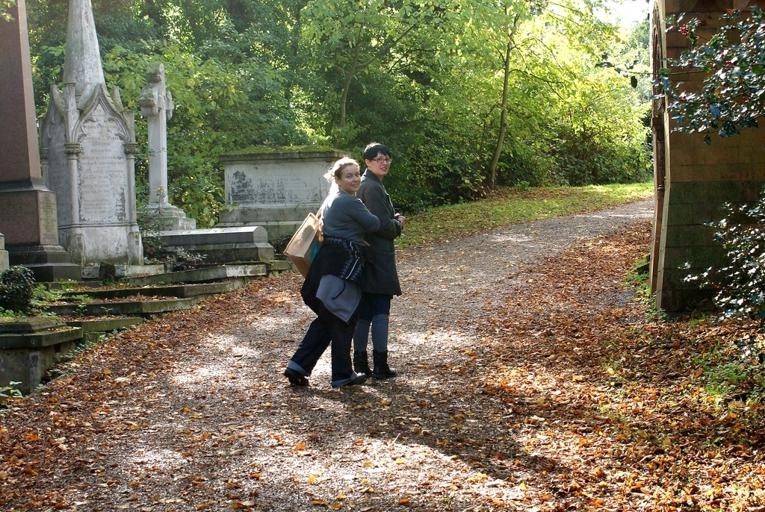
[284,157,381,389]
[356,142,409,381]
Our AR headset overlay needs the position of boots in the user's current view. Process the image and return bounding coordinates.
[371,348,398,380]
[352,348,373,380]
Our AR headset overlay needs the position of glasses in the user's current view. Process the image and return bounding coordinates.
[368,157,393,164]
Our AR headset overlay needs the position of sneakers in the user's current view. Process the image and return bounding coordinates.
[329,372,367,389]
[282,367,310,386]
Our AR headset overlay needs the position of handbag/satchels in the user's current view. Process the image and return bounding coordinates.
[284,211,324,279]
